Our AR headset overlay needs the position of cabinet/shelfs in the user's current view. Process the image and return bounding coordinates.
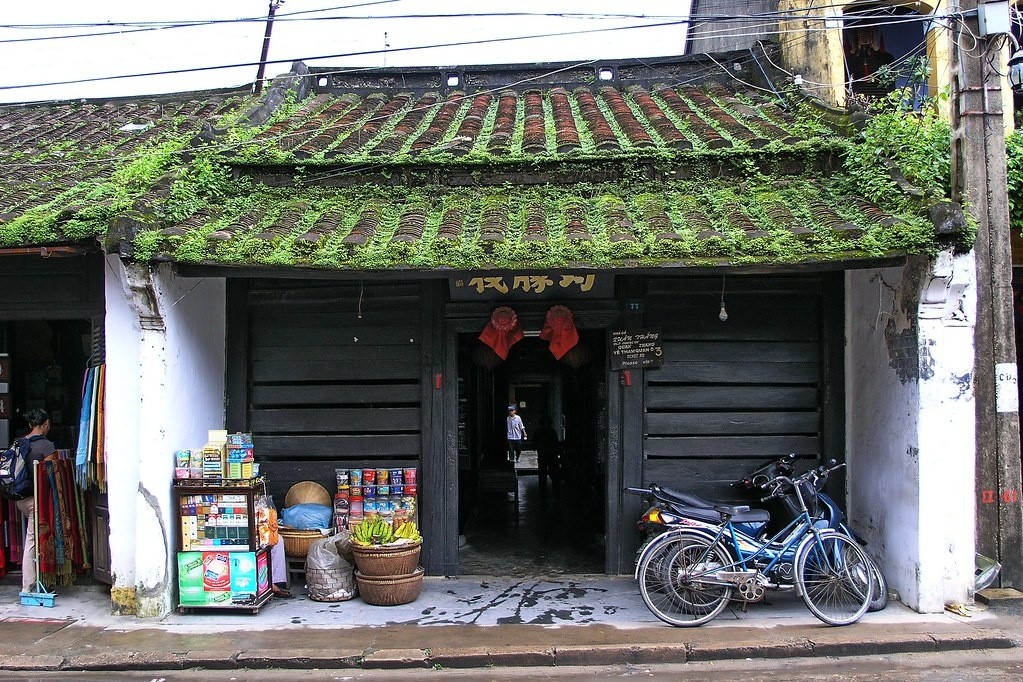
[173,474,273,614]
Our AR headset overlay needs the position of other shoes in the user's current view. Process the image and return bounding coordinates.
[40,588,55,594]
[516,457,520,463]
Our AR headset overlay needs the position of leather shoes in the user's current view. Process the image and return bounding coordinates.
[272,590,290,597]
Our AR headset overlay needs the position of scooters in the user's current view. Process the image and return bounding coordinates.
[622,451,889,615]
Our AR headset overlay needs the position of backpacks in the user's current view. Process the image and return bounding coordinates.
[0,435,46,501]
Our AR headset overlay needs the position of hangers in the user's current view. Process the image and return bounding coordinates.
[87,339,106,368]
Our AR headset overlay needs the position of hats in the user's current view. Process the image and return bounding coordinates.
[508,406,516,411]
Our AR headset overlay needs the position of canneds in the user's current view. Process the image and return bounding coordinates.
[372,534,380,545]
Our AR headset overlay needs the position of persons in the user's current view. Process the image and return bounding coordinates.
[507,406,527,463]
[532,413,559,499]
[15,409,57,592]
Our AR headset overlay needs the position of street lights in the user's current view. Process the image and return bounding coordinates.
[957,32,1023,602]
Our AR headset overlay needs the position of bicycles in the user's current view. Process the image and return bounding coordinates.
[635,463,872,628]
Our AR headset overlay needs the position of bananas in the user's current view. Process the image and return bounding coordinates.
[348,517,421,546]
